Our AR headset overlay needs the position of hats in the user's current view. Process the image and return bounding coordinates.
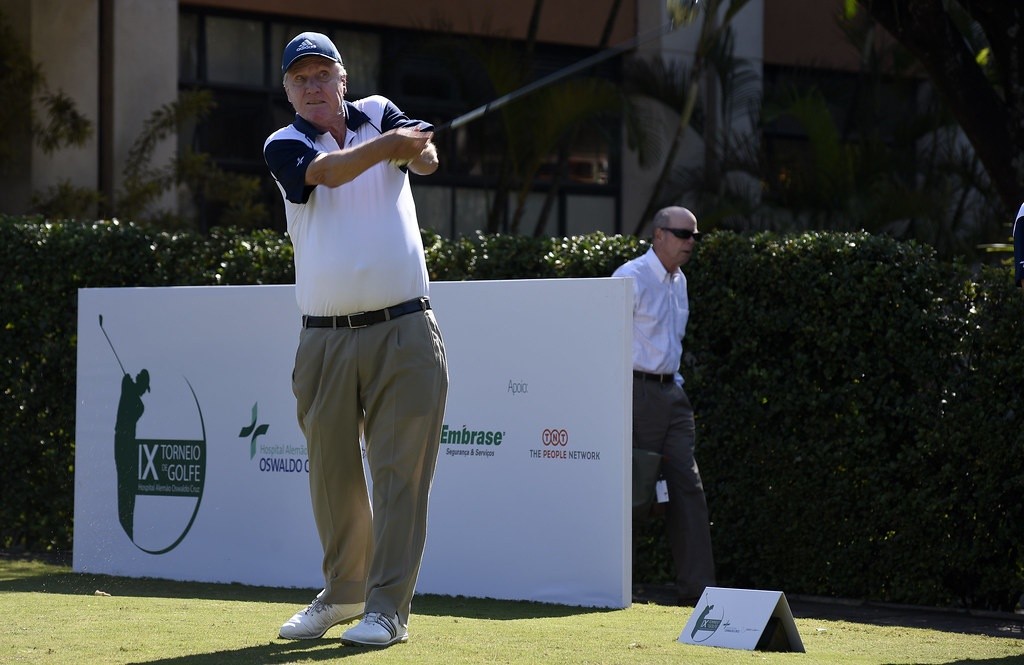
[281,31,344,74]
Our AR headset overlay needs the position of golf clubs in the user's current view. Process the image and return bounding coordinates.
[431,0,697,138]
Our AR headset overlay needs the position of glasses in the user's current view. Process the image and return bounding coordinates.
[660,228,703,242]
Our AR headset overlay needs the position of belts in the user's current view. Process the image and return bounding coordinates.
[633,370,674,384]
[302,299,430,328]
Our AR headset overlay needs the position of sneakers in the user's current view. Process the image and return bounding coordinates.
[340,612,409,648]
[278,598,366,640]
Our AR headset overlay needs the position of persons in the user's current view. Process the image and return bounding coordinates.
[263,31,448,647]
[611,206,717,607]
